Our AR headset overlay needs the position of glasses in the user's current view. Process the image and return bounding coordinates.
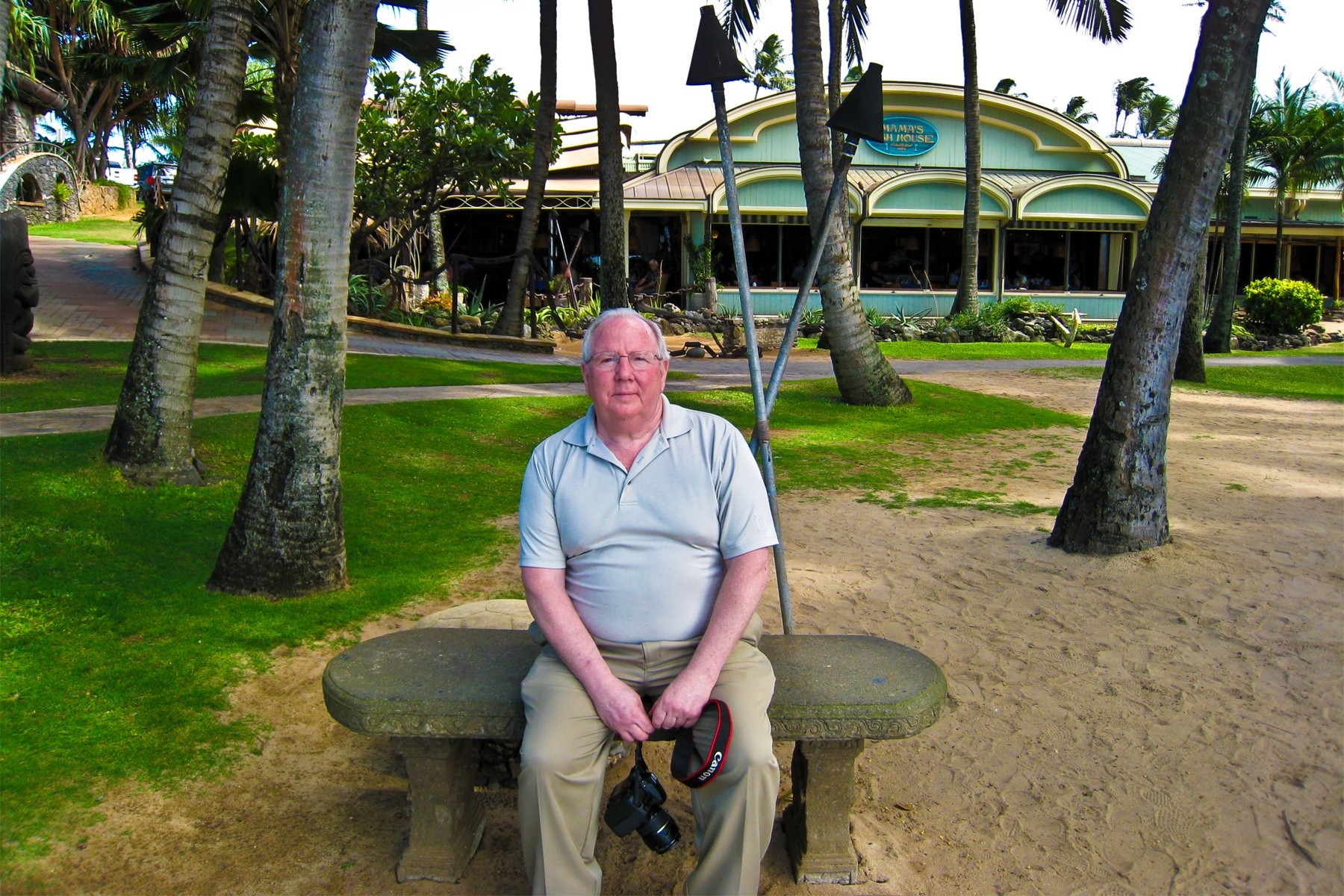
[586,350,661,371]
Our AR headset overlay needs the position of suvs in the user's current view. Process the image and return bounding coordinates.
[141,161,178,200]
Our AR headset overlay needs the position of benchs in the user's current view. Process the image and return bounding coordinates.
[320,626,945,885]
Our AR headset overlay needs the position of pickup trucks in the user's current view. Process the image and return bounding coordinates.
[94,161,140,188]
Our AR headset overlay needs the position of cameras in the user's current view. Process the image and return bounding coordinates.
[603,743,682,856]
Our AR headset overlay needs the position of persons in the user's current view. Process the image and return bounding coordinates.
[949,267,961,289]
[1009,268,1030,290]
[720,264,739,287]
[559,260,578,290]
[866,260,892,289]
[633,260,660,303]
[519,310,779,896]
